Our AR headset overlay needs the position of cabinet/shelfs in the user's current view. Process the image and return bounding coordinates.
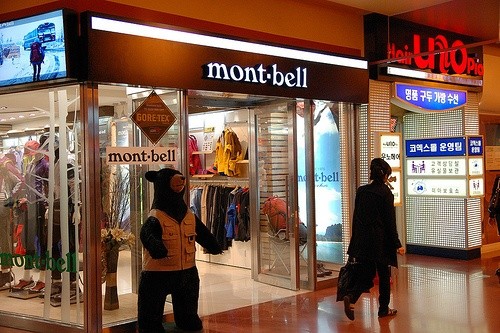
[185,107,252,269]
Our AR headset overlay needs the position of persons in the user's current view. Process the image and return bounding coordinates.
[343,157,405,321]
[0,132,83,303]
[29,37,45,81]
[487,175,500,276]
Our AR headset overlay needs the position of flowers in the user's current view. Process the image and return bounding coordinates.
[100,166,144,251]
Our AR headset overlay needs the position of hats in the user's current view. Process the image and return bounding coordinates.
[23,141,41,156]
[38,132,59,149]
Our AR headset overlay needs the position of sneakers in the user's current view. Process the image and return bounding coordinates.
[387,307,397,316]
[344,296,355,320]
[54,283,82,302]
[0,271,13,287]
[29,280,45,294]
[12,276,35,292]
[38,282,62,298]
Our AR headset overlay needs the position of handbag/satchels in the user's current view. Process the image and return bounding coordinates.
[336,256,371,302]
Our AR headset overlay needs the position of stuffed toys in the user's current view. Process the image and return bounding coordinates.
[137,168,223,333]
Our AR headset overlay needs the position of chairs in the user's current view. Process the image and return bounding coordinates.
[265,214,306,275]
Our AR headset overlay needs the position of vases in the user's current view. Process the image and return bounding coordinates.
[104,248,120,311]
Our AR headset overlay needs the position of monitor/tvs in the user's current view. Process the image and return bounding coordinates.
[0,9,67,88]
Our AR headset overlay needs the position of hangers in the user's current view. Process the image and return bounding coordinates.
[187,180,249,196]
[189,124,239,140]
